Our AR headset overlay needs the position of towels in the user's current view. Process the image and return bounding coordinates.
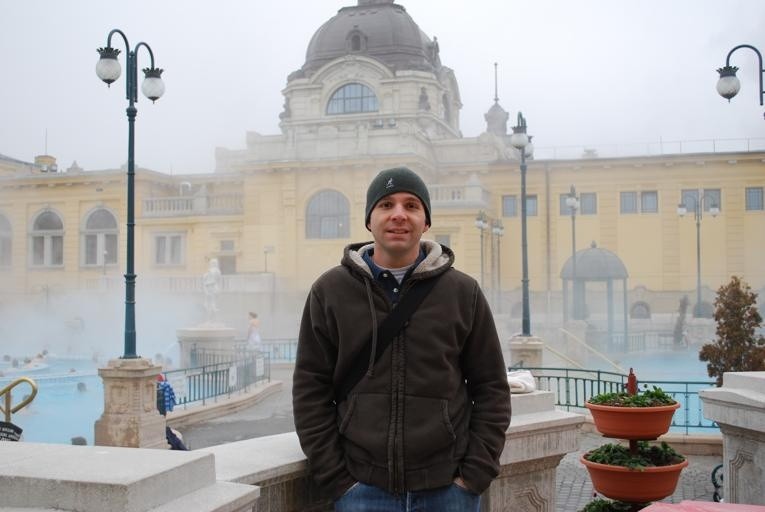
[505,368,537,394]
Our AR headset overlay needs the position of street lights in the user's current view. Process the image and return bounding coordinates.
[491,215,504,317]
[508,110,542,368]
[566,184,582,318]
[716,39,765,140]
[476,208,488,295]
[94,29,170,451]
[677,194,722,320]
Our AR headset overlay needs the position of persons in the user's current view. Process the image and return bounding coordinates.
[245,312,260,358]
[419,87,430,110]
[0,349,48,377]
[164,358,174,370]
[292,166,512,512]
[77,382,86,393]
[431,36,439,61]
[71,437,86,445]
[203,258,222,313]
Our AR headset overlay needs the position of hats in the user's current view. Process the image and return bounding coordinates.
[363,167,431,232]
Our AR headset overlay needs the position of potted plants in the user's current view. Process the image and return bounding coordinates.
[580,438,690,502]
[584,383,681,440]
